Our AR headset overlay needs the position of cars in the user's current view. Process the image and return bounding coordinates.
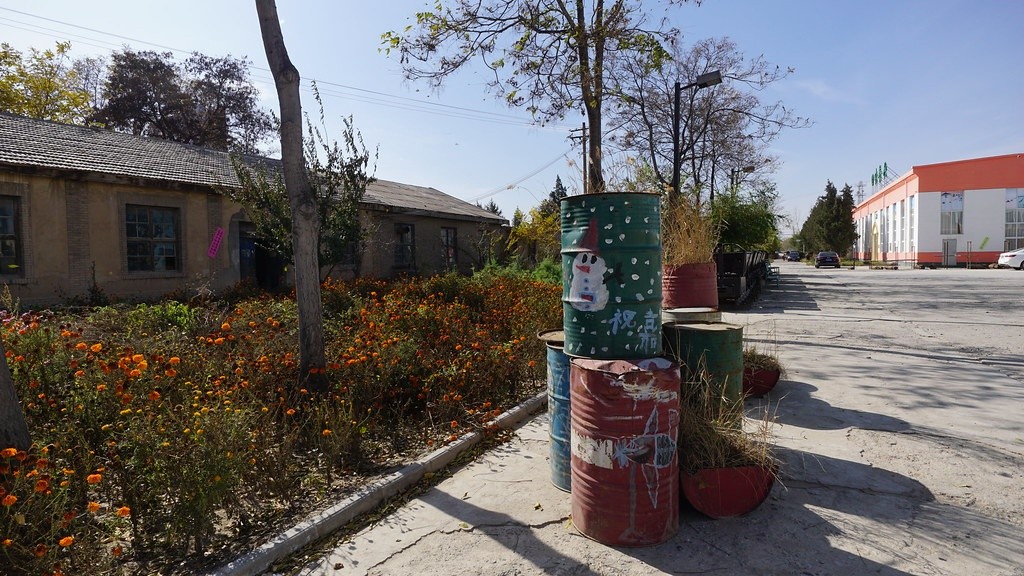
[779,250,801,262]
[996,245,1024,270]
[815,251,842,269]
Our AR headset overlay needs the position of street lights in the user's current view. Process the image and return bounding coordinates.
[672,69,724,194]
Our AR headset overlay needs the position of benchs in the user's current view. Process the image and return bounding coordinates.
[762,259,781,288]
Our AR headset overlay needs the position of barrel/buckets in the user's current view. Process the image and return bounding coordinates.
[570,354,680,545]
[546,341,571,493]
[661,306,722,322]
[559,191,663,360]
[662,321,744,435]
[661,260,718,309]
